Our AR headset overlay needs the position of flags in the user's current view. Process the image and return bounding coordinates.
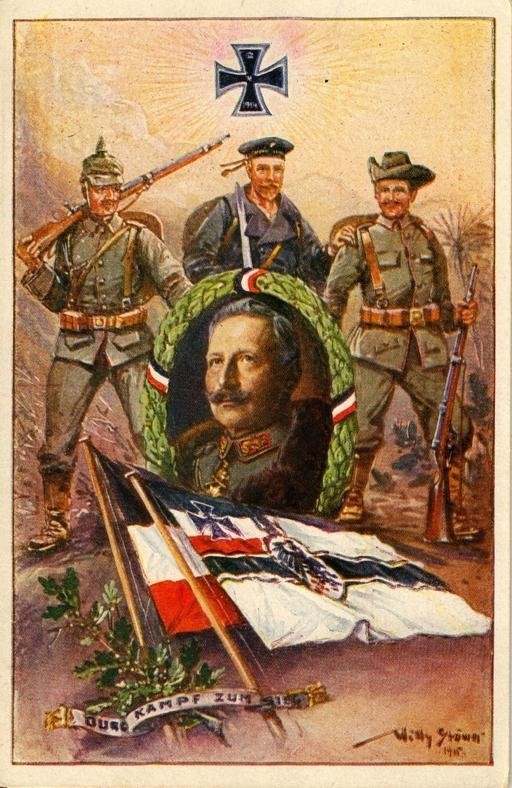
[135,474,492,652]
[94,446,247,637]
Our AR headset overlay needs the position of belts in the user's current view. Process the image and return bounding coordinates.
[64,313,148,329]
[358,306,439,328]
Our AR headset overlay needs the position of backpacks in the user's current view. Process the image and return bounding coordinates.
[182,201,216,281]
[120,209,163,303]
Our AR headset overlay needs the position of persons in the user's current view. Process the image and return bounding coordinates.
[321,150,485,545]
[171,294,333,517]
[181,135,357,299]
[16,135,195,555]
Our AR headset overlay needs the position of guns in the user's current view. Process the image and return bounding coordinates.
[18,134,229,256]
[424,264,481,544]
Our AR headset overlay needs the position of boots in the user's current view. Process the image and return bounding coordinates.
[339,448,376,522]
[436,450,485,542]
[30,471,70,556]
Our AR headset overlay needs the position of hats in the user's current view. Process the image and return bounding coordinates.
[238,138,293,158]
[367,152,436,189]
[80,154,125,187]
